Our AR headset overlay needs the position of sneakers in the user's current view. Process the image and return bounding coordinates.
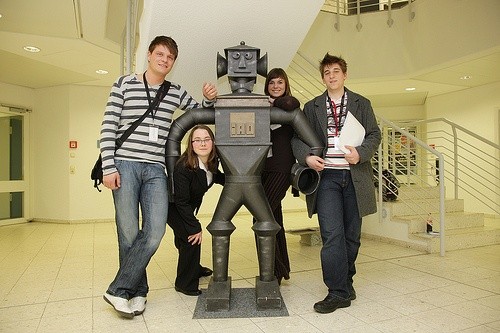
[129,296,147,316]
[348,286,356,300]
[103,293,134,319]
[314,294,351,313]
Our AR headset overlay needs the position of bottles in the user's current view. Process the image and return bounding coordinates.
[427,213,433,233]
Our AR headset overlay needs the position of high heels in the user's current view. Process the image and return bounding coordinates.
[277,271,290,286]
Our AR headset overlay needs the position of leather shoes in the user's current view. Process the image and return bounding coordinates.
[202,267,212,276]
[174,286,202,296]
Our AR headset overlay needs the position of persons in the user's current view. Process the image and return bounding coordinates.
[291,53,382,314]
[252,68,300,284]
[166,126,226,297]
[100,36,217,318]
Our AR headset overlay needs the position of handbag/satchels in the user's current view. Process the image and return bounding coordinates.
[91,153,103,192]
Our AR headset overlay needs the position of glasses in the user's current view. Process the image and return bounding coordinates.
[192,138,212,145]
